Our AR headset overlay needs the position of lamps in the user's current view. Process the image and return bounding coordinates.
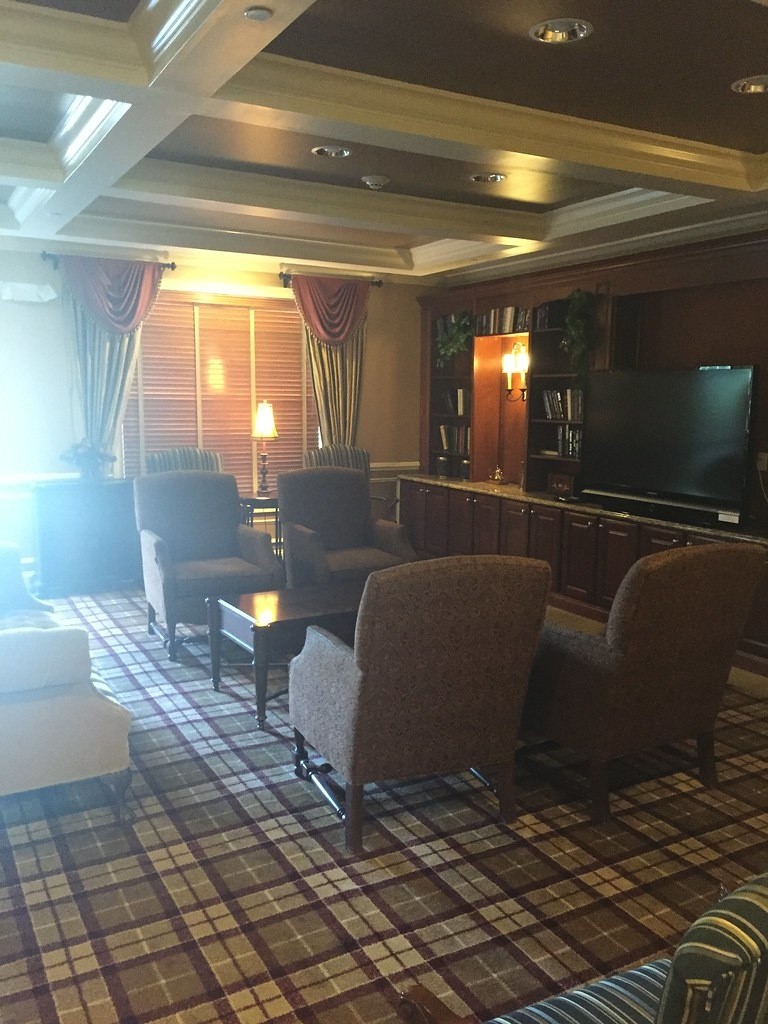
[249,398,278,496]
[501,342,529,402]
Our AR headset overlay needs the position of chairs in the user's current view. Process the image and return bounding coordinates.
[302,444,399,524]
[287,554,551,853]
[276,467,417,588]
[517,543,767,826]
[143,447,252,527]
[133,469,283,662]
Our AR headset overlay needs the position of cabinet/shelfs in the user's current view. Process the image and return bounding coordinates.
[394,230,768,680]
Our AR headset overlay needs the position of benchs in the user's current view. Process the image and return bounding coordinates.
[394,871,768,1024]
[0,540,139,828]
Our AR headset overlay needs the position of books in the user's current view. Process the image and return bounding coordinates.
[437,425,471,455]
[531,300,569,331]
[440,387,470,416]
[540,387,582,422]
[474,305,529,336]
[537,424,582,460]
[434,313,459,339]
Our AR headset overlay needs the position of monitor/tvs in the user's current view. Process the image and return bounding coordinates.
[582,366,757,533]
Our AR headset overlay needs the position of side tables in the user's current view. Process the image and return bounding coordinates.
[240,497,283,560]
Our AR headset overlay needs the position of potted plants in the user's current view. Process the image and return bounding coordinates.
[58,436,118,481]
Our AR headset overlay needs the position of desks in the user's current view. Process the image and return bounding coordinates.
[204,578,366,731]
[36,478,142,597]
